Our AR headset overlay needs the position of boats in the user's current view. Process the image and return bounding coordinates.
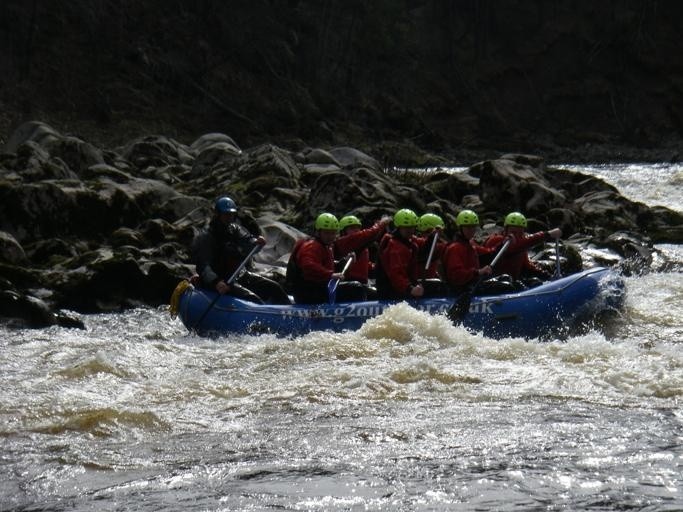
[177,268,627,342]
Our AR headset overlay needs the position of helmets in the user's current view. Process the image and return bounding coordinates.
[217,197,237,214]
[315,213,338,231]
[504,212,527,227]
[417,213,443,231]
[393,209,418,226]
[339,216,361,231]
[456,210,478,226]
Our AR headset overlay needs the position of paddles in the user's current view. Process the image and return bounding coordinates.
[327,257,352,305]
[451,240,511,322]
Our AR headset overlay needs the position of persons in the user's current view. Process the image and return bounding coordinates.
[192,196,293,306]
[478,213,563,292]
[374,208,445,304]
[335,215,375,303]
[293,213,393,305]
[416,212,448,280]
[441,209,517,297]
